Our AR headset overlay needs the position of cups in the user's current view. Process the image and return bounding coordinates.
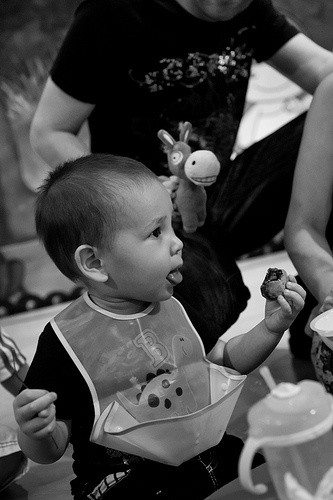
[309,309,333,350]
[238,381,332,500]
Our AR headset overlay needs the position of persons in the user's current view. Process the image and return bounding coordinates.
[29,0,333,311]
[11,154,307,500]
[285,73,333,332]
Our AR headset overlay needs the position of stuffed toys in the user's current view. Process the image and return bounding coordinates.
[145,120,223,235]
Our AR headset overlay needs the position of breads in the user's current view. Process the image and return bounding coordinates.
[260,267,289,300]
